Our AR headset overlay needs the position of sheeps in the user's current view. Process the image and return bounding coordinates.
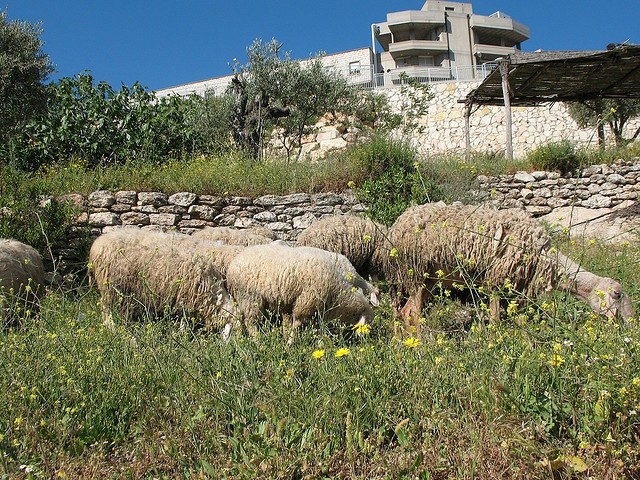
[225,241,382,357]
[294,213,389,283]
[376,199,637,350]
[192,227,276,247]
[88,227,252,350]
